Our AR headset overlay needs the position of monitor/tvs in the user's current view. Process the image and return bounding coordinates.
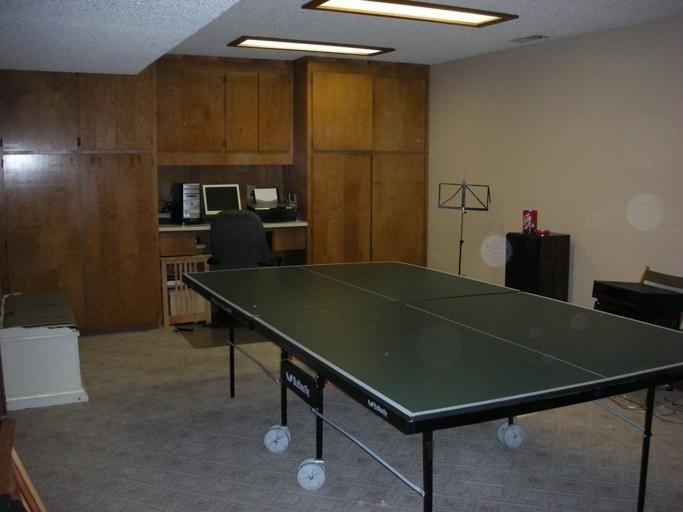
[204,184,242,219]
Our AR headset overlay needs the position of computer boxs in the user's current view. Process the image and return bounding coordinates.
[173,181,203,223]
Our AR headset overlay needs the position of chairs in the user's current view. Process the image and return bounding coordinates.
[204,209,285,271]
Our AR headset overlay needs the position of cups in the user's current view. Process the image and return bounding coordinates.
[522,210,538,234]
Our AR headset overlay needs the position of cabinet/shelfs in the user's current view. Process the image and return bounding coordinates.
[80,153,160,325]
[0,290,89,411]
[505,230,570,304]
[225,70,291,154]
[156,70,225,152]
[591,279,683,331]
[292,61,428,153]
[0,69,78,154]
[284,155,429,269]
[78,73,156,152]
[0,155,79,328]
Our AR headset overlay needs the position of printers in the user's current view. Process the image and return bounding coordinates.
[248,188,298,223]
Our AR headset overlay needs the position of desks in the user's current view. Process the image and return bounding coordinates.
[181,260,682,511]
[159,220,307,329]
